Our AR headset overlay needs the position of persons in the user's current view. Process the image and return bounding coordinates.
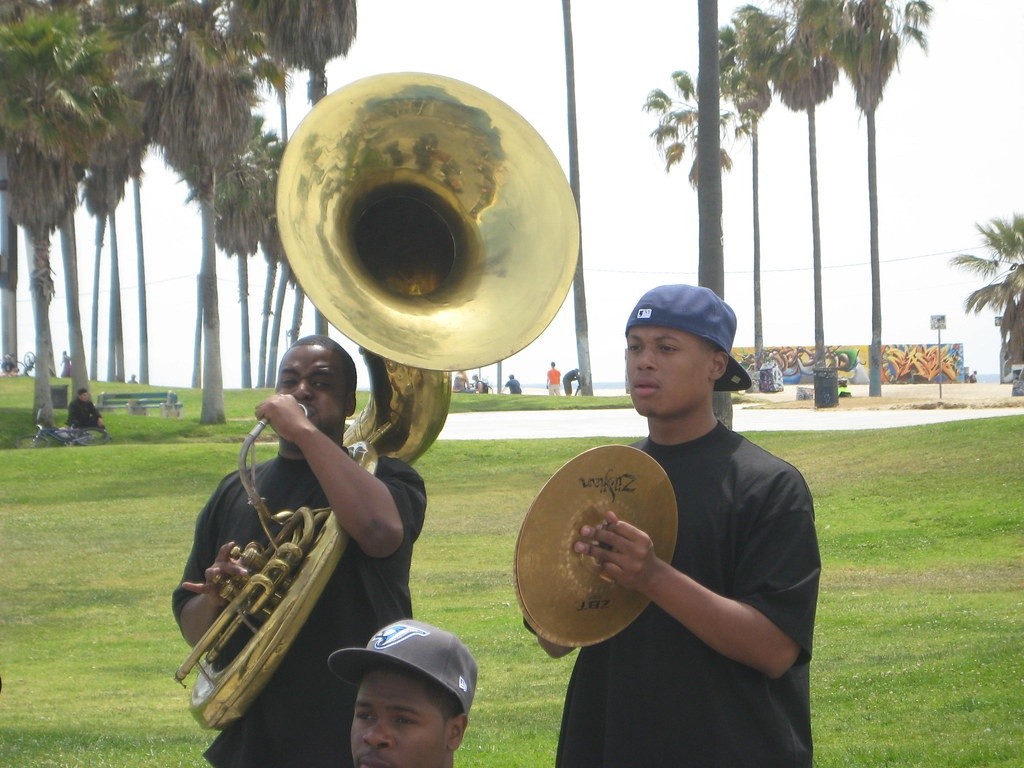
[128,375,138,384]
[67,387,112,440]
[547,361,561,395]
[1,354,17,377]
[171,334,427,768]
[502,375,522,394]
[327,619,478,768]
[970,371,977,382]
[60,351,70,377]
[452,371,488,393]
[519,284,823,768]
[563,368,578,396]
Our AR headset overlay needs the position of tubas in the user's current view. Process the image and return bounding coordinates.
[175,75,578,731]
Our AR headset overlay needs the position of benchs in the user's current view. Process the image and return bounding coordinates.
[95,391,184,418]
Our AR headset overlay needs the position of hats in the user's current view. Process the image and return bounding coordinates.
[626,284,752,392]
[328,620,478,716]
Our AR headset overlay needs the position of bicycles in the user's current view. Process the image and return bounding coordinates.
[16,403,111,449]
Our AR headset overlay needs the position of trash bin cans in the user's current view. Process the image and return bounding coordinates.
[812,367,839,407]
[50,385,69,409]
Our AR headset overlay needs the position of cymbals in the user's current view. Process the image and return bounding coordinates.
[513,444,678,647]
